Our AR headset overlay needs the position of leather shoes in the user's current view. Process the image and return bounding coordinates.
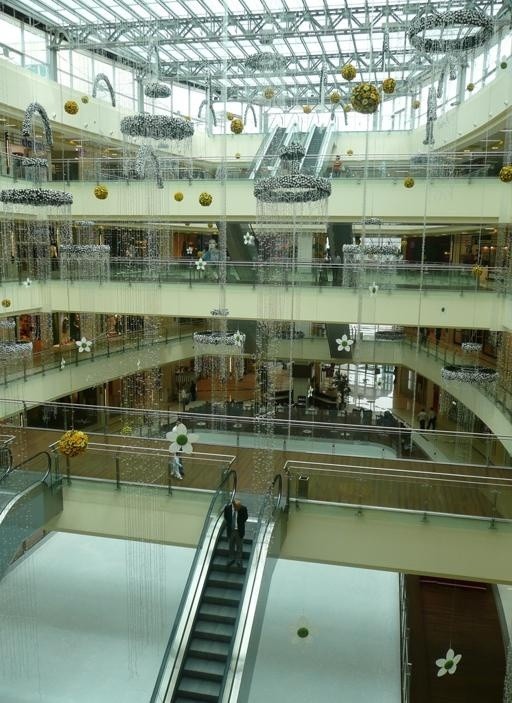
[237,559,242,566]
[227,559,235,566]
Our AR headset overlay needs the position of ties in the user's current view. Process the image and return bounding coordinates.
[230,508,236,531]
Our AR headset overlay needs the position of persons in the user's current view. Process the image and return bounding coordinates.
[415,408,428,432]
[73,314,81,342]
[426,407,437,430]
[50,245,59,271]
[172,419,185,479]
[18,314,33,342]
[224,498,248,567]
[62,317,70,343]
[420,327,430,349]
[332,154,342,178]
